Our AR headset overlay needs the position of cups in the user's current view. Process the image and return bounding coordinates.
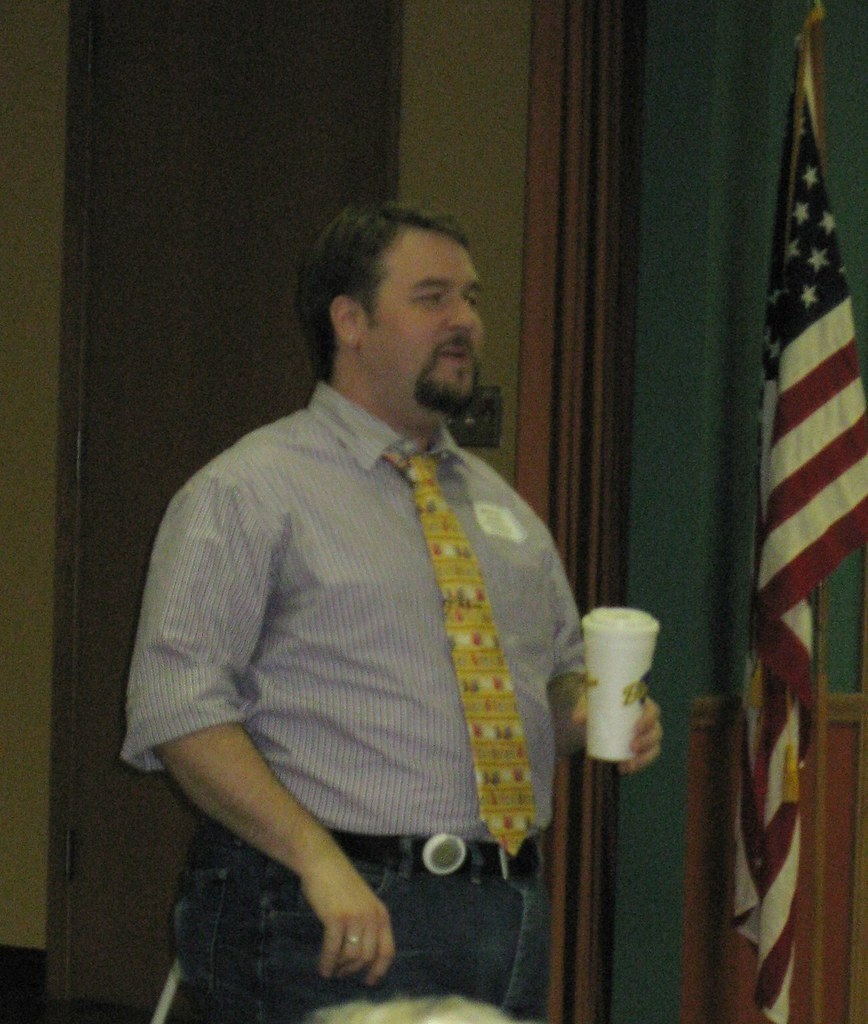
[580,605,659,763]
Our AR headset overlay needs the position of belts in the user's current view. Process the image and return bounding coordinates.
[337,828,535,877]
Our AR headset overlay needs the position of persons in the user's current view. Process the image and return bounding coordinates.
[120,202,662,1024]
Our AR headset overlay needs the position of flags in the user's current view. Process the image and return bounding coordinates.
[735,8,868,1024]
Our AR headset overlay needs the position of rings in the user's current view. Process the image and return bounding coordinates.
[345,935,361,944]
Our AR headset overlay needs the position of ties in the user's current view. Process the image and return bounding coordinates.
[384,447,536,859]
